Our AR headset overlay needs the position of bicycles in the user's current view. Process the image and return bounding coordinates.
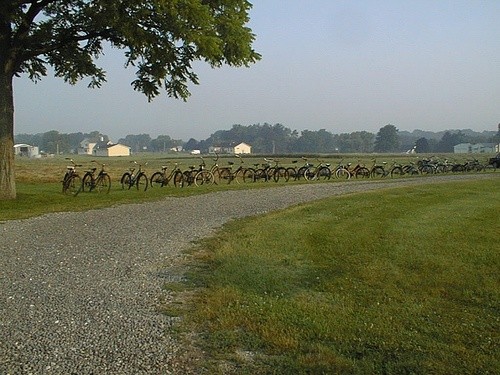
[150,160,184,188]
[120,160,149,192]
[188,153,232,186]
[217,154,500,186]
[180,155,214,187]
[60,158,83,197]
[82,160,111,195]
